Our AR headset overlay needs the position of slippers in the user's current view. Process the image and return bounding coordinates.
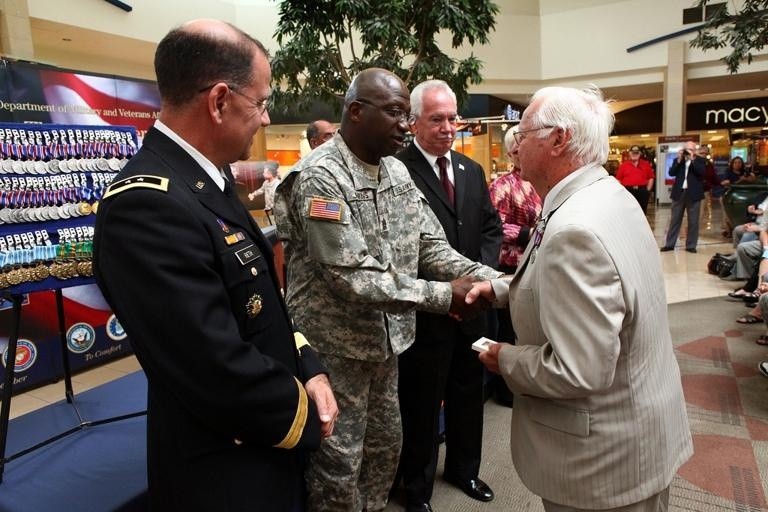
[756,334,768,345]
[736,313,764,323]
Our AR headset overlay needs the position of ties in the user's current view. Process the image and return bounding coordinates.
[222,176,256,230]
[434,156,455,208]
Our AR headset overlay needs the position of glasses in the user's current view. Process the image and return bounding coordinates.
[198,84,273,115]
[513,126,553,146]
[359,98,417,125]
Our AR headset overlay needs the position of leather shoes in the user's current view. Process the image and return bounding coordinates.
[412,502,433,512]
[729,288,758,303]
[443,471,494,501]
[686,248,696,253]
[661,247,674,251]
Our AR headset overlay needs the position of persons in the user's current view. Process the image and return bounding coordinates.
[91,18,339,512]
[248,163,281,210]
[392,79,504,512]
[488,124,541,409]
[660,141,768,378]
[273,68,509,512]
[615,145,656,215]
[307,120,336,150]
[449,85,694,512]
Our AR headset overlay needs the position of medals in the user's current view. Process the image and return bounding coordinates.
[0,156,130,290]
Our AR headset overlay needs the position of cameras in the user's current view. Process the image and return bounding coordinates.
[743,167,752,177]
[683,149,690,156]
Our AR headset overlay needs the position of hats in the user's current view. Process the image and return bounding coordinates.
[629,146,640,152]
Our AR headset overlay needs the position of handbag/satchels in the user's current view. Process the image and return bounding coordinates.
[707,252,738,277]
